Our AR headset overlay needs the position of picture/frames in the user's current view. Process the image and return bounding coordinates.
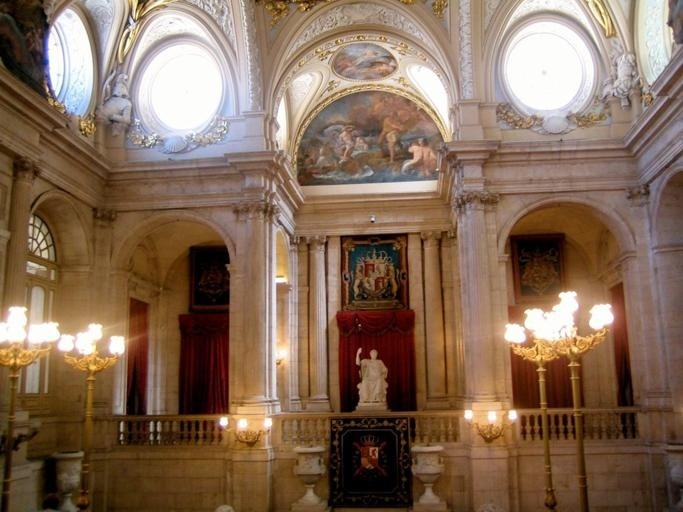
[189,245,230,314]
[509,232,567,304]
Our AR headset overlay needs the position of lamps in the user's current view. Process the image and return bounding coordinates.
[219,417,273,446]
[463,410,518,443]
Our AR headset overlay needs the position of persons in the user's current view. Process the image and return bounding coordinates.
[355,346,389,402]
[300,111,439,182]
